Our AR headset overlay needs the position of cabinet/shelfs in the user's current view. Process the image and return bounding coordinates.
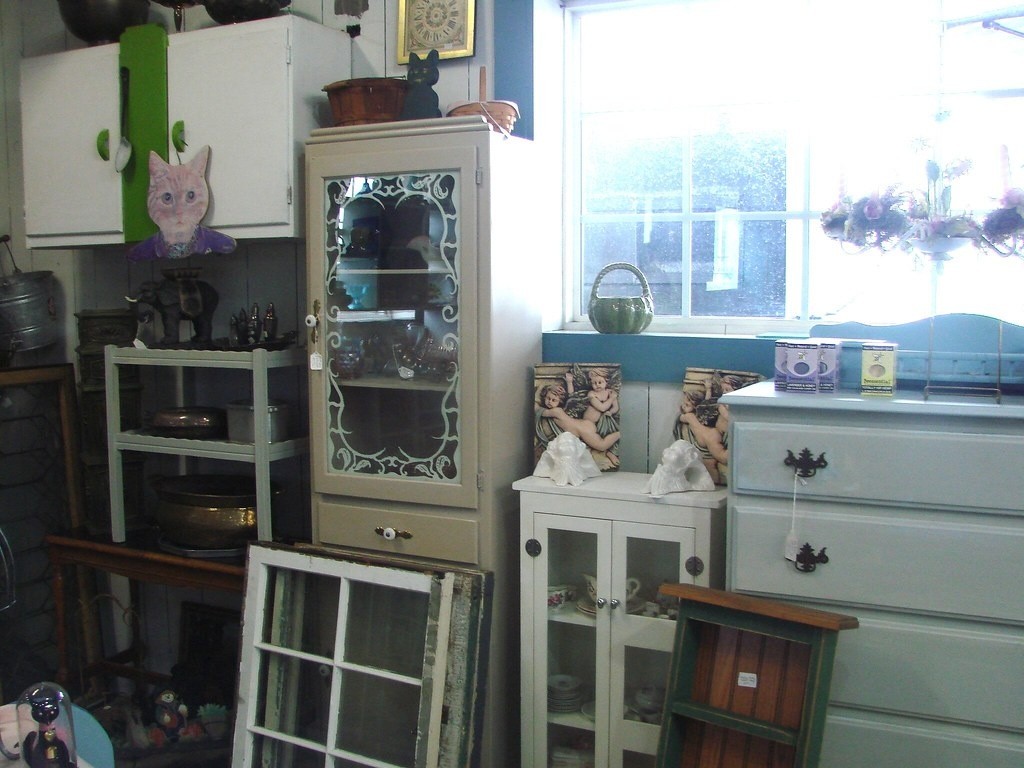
[726,398,1023,768]
[304,114,563,768]
[20,14,352,252]
[512,470,729,768]
[104,342,304,702]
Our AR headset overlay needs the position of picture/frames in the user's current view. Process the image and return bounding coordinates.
[397,0,475,65]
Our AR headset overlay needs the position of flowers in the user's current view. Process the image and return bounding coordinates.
[819,185,1023,260]
